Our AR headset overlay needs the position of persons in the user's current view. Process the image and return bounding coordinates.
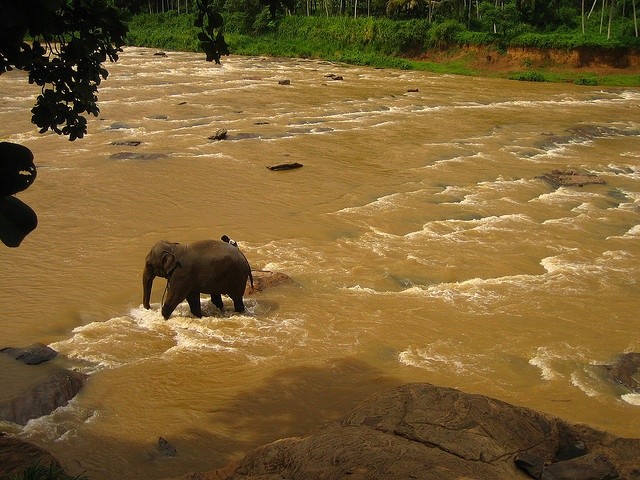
[221,234,238,246]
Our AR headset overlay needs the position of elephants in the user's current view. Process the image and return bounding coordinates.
[142,239,254,321]
